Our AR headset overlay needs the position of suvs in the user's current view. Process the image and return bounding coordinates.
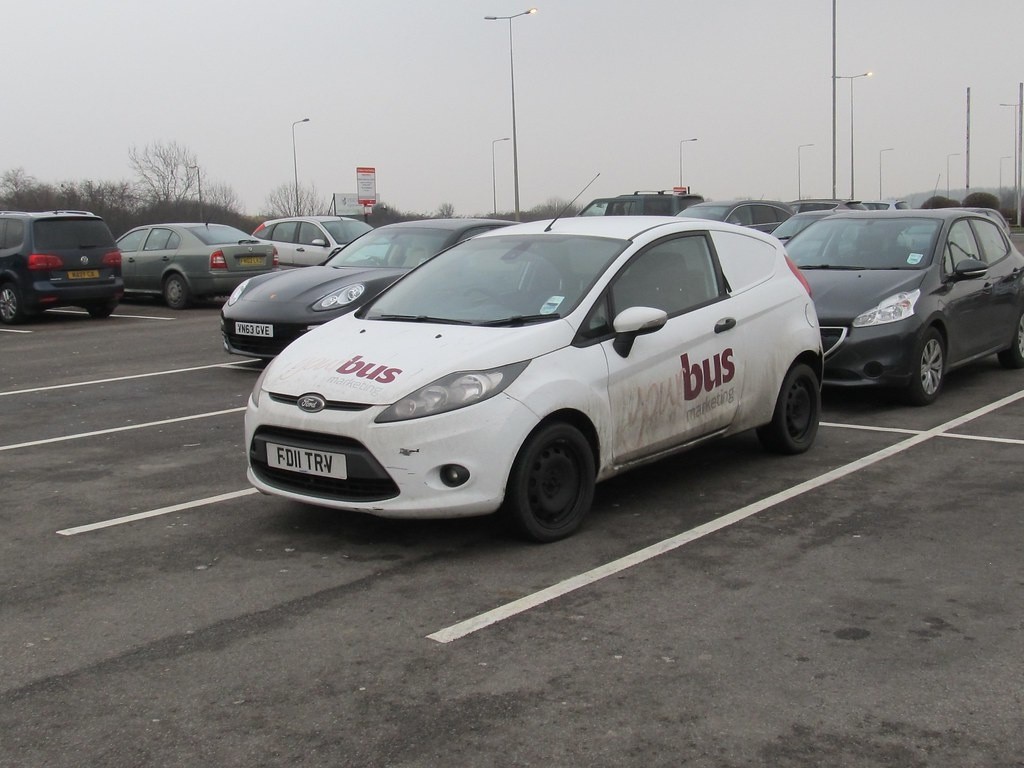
[0,209,124,326]
[573,189,705,217]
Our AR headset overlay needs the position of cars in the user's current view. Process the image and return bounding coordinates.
[776,209,1023,407]
[112,222,278,310]
[252,216,397,273]
[676,199,795,235]
[216,218,519,356]
[769,197,909,250]
[898,207,1011,256]
[242,214,823,544]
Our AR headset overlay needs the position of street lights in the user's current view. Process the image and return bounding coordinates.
[999,156,1013,196]
[484,9,537,220]
[189,165,204,223]
[680,138,698,188]
[831,73,871,202]
[879,148,895,201]
[491,138,510,218]
[946,153,960,199]
[999,103,1018,219]
[797,144,815,198]
[291,118,313,214]
